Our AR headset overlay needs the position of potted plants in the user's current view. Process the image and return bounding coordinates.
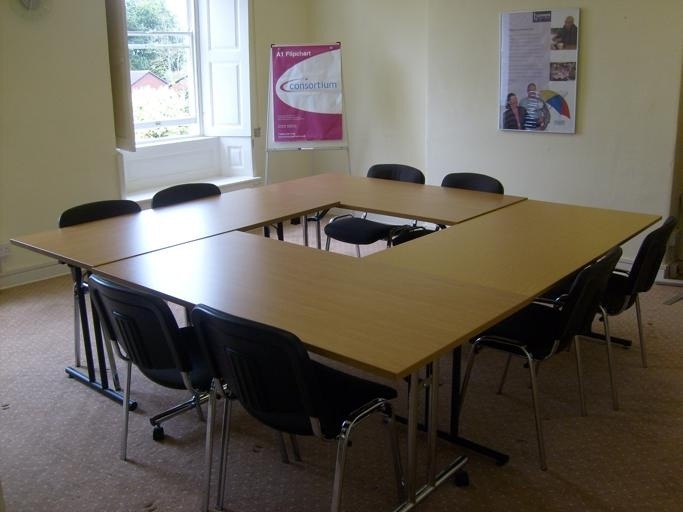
[496,7,580,134]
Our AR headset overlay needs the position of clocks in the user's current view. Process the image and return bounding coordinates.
[7,0,55,22]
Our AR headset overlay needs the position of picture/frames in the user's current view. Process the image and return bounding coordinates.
[496,7,580,134]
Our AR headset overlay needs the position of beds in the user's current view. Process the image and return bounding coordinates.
[539,89,570,119]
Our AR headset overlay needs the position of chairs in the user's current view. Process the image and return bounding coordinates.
[6,161,678,511]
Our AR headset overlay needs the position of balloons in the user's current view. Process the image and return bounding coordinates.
[7,0,55,22]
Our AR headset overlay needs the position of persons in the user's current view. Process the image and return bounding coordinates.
[518,83,549,130]
[502,93,529,129]
[552,16,577,50]
